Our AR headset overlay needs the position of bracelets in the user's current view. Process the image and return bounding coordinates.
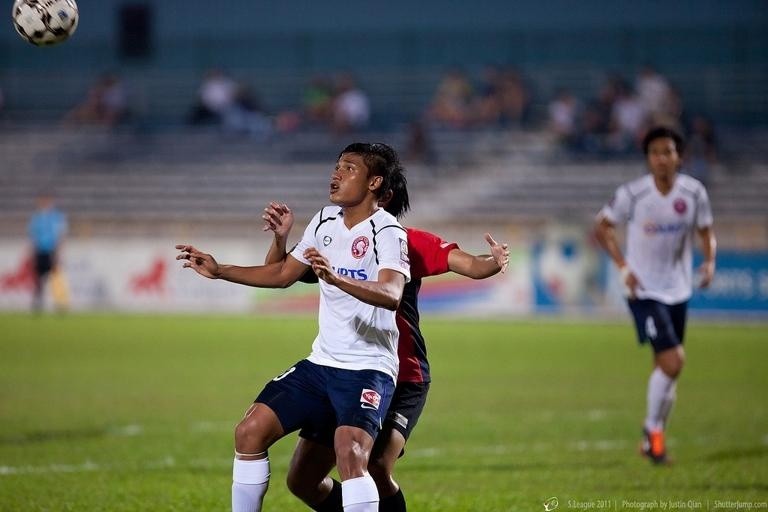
[620,264,630,282]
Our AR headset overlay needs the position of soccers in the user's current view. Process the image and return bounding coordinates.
[12,0,79,46]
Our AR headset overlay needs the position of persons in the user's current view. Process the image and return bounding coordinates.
[263,171,510,512]
[24,193,69,316]
[176,142,412,512]
[594,125,716,464]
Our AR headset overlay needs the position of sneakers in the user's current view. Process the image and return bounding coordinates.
[640,421,671,461]
